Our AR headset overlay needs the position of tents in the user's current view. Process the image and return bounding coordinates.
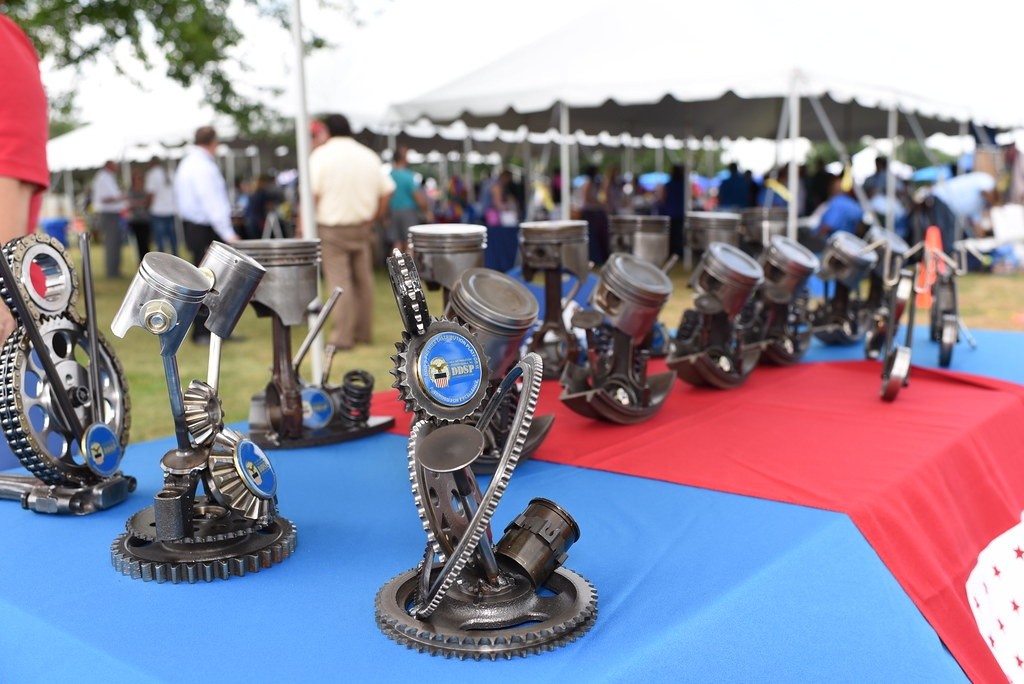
[47,77,240,246]
[385,0,1024,280]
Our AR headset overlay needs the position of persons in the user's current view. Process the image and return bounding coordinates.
[297,111,398,350]
[234,147,930,240]
[92,160,131,277]
[131,156,177,257]
[924,170,994,254]
[176,126,241,344]
[0,12,51,350]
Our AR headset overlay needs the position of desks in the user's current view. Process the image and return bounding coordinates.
[0,316,1024,684]
